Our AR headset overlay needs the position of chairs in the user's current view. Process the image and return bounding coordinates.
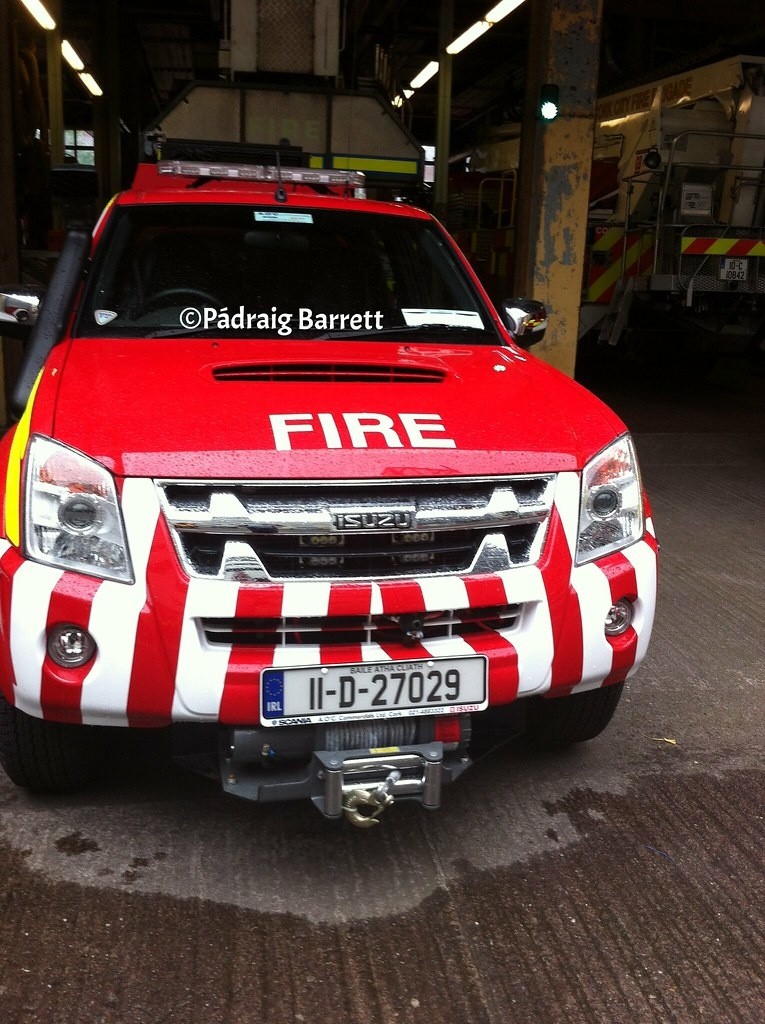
[119,231,271,322]
[274,246,386,320]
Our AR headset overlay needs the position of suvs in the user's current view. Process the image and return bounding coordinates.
[0,151,657,829]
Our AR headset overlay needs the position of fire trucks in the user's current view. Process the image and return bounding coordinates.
[445,55,765,373]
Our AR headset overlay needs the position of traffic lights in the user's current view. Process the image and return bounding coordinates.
[541,82,559,121]
